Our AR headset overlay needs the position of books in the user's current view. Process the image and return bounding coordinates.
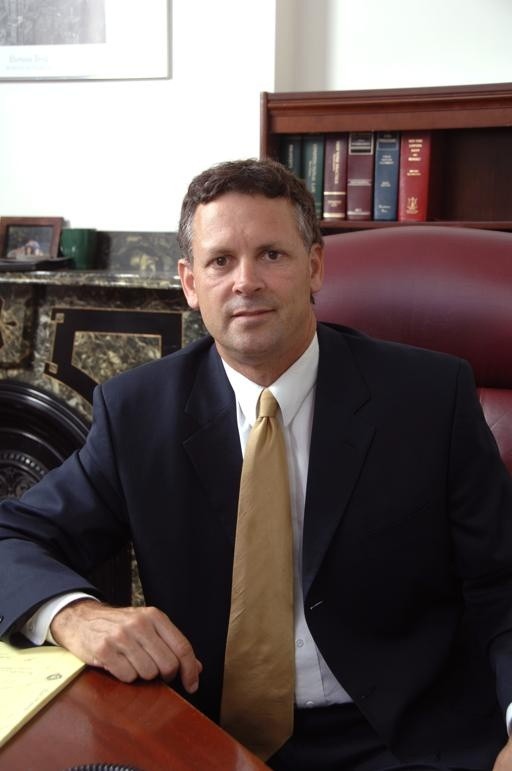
[295,136,323,220]
[322,137,349,219]
[398,132,430,223]
[317,135,348,222]
[283,135,300,181]
[373,132,398,221]
[347,133,374,223]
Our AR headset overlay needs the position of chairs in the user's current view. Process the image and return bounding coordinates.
[310,225,510,463]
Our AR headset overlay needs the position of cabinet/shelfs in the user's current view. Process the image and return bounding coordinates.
[259,84,512,243]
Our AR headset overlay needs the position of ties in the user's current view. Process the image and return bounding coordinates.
[222,389,295,764]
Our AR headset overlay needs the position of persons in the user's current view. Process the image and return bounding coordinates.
[0,157,512,770]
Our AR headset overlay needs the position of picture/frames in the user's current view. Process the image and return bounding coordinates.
[0,215,63,266]
